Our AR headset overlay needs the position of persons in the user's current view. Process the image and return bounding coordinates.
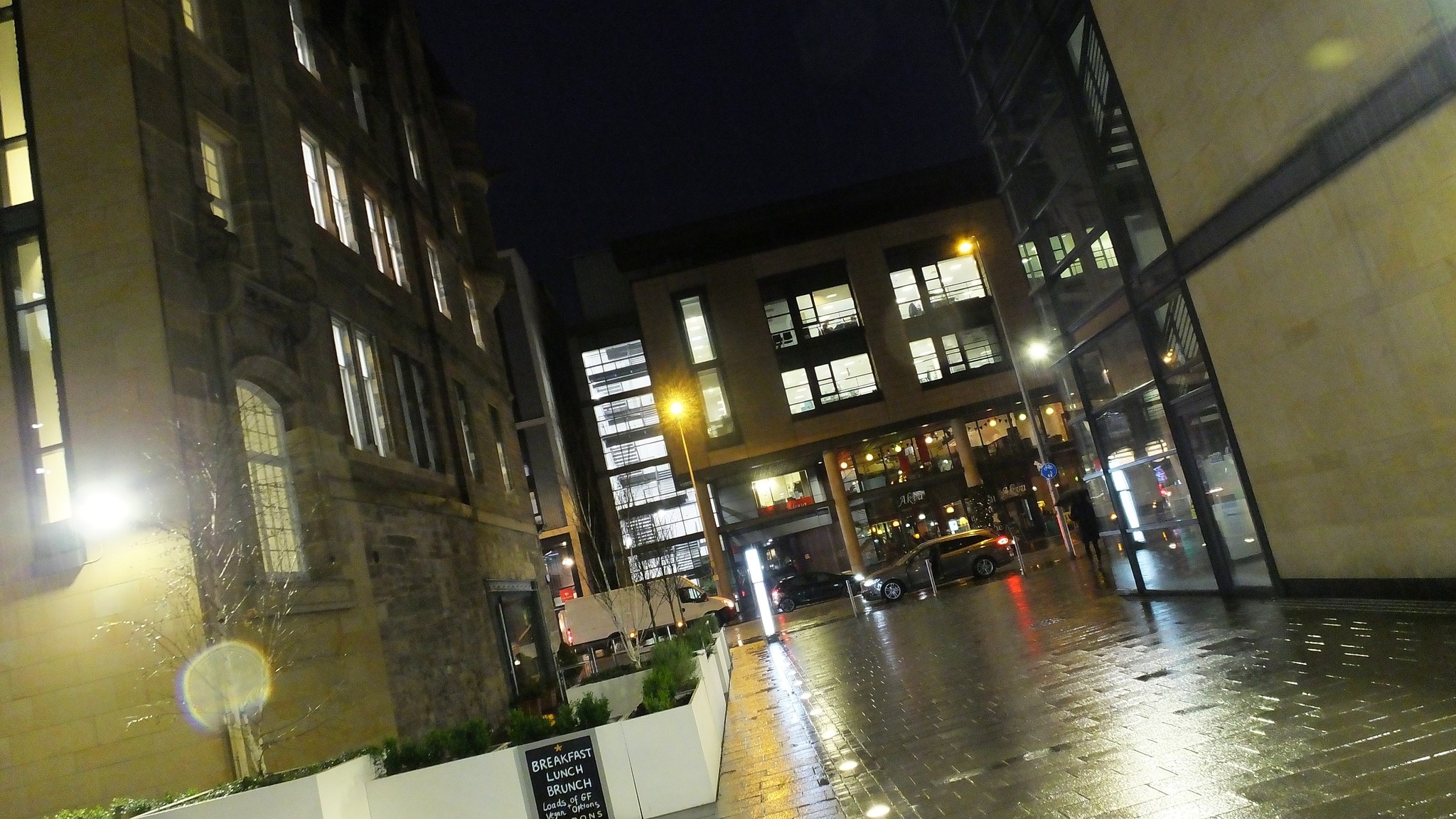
[1070,495,1102,562]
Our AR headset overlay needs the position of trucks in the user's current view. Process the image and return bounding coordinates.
[563,576,740,654]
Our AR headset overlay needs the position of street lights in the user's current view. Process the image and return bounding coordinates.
[955,235,1078,555]
[665,393,734,600]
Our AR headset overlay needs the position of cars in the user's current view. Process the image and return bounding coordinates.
[861,529,1015,606]
[771,571,861,613]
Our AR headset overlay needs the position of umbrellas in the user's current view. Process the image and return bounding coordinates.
[1055,487,1089,507]
